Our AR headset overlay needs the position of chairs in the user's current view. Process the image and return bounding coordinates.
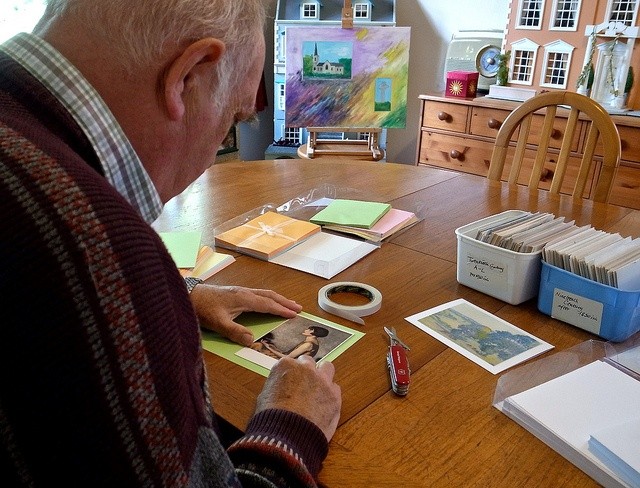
[486,91,622,203]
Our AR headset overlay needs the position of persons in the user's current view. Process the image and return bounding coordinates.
[260,326,331,357]
[1,0,342,488]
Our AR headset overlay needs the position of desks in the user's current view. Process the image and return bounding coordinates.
[148,157,640,488]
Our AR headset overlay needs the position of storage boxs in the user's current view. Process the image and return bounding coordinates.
[455,209,543,305]
[538,257,640,343]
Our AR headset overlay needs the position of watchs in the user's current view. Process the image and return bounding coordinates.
[184,276,204,293]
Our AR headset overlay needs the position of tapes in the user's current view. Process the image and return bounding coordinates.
[317,283,383,326]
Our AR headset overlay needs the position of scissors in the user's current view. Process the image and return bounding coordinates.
[383,326,411,351]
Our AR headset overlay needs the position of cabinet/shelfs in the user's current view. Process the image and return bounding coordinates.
[415,90,640,209]
